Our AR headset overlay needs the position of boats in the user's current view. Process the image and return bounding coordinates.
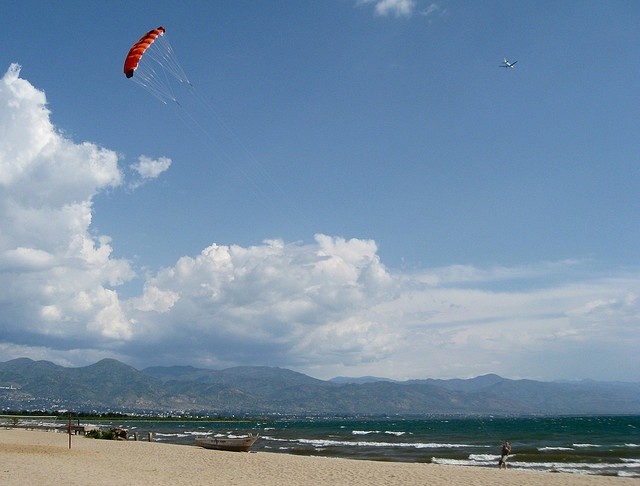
[195,433,261,452]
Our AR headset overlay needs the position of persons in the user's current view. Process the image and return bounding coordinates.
[499,440,512,468]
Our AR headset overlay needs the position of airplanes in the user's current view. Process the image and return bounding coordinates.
[499,57,518,70]
